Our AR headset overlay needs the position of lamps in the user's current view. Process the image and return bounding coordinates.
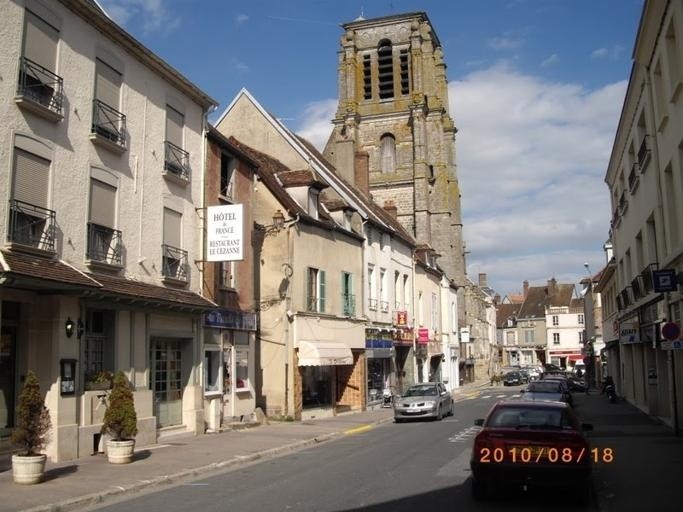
[272,209,285,232]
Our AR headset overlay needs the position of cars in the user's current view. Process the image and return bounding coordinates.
[394,382,454,421]
[468,364,594,500]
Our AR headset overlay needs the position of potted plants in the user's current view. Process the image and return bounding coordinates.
[100,368,138,464]
[9,367,54,485]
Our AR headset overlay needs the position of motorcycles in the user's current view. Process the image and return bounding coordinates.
[602,375,617,401]
